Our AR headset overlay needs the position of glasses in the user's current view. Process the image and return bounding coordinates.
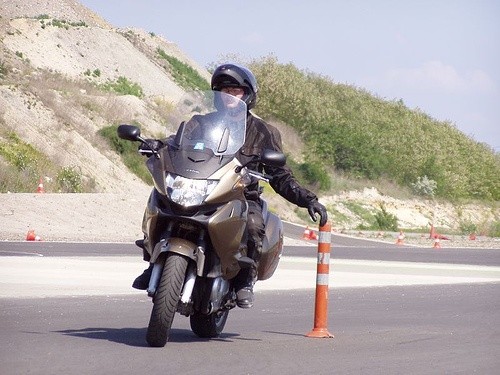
[220,88,243,94]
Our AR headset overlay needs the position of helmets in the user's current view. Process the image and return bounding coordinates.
[210,63,259,116]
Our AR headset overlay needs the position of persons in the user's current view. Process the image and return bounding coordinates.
[132,64,327,309]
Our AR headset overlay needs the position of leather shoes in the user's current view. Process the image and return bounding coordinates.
[235,286,254,308]
[132,268,153,290]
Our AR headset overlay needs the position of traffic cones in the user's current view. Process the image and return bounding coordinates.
[432,236,440,248]
[309,231,319,239]
[37,176,45,194]
[396,232,404,245]
[301,226,309,240]
[26,229,43,241]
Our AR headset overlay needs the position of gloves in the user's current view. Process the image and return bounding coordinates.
[308,199,328,227]
[138,139,164,158]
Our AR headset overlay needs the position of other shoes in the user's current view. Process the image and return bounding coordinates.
[135,239,146,249]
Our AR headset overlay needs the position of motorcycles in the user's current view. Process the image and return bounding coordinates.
[117,91,284,348]
